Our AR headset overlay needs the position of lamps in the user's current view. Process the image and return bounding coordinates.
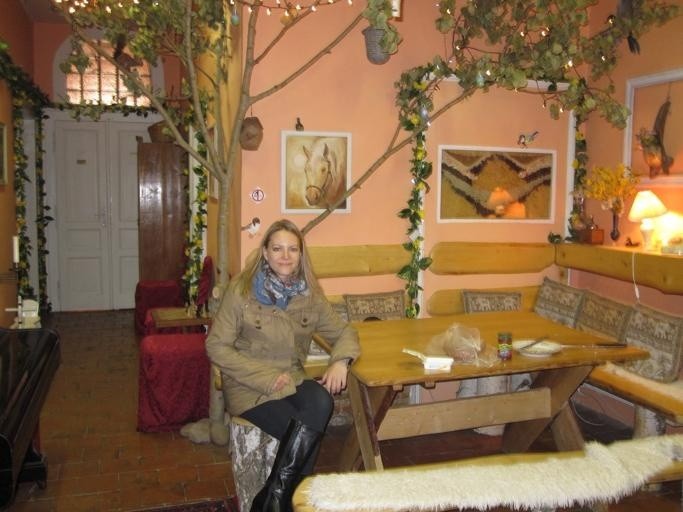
[629,190,667,248]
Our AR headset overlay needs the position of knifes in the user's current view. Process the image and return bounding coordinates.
[558,343,627,349]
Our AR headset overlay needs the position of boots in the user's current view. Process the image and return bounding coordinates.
[249,416,321,512]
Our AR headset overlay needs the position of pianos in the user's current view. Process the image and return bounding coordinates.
[0,325,62,509]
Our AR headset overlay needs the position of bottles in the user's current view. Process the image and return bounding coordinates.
[495,331,513,361]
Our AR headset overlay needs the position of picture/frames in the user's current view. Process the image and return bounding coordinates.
[280,129,353,215]
[0,121,8,187]
[622,70,682,187]
[435,144,556,223]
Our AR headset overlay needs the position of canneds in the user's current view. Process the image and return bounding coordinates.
[498,333,512,361]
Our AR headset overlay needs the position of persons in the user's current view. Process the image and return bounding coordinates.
[202,220,360,512]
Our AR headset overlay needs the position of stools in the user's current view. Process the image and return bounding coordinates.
[137,332,213,433]
[134,279,186,330]
[228,418,321,512]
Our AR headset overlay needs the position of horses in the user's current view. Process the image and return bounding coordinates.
[303,137,346,209]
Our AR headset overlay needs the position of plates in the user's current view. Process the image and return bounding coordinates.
[510,340,563,358]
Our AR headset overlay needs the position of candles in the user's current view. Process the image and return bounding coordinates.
[12,236,19,262]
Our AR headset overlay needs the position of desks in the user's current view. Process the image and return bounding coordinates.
[152,306,212,332]
